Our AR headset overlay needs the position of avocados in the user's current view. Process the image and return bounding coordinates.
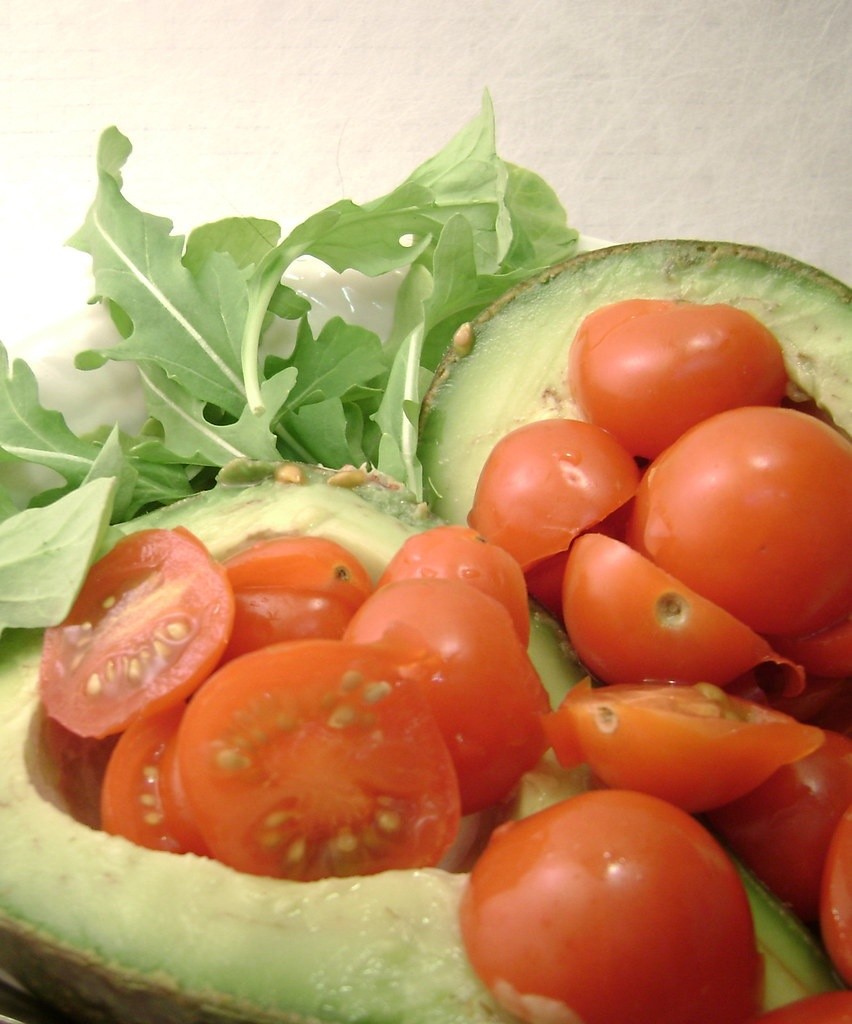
[415,235,851,530]
[1,453,852,1024]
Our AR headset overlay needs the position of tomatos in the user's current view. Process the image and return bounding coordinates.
[38,301,852,1024]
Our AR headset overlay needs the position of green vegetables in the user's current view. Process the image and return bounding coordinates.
[0,83,586,640]
